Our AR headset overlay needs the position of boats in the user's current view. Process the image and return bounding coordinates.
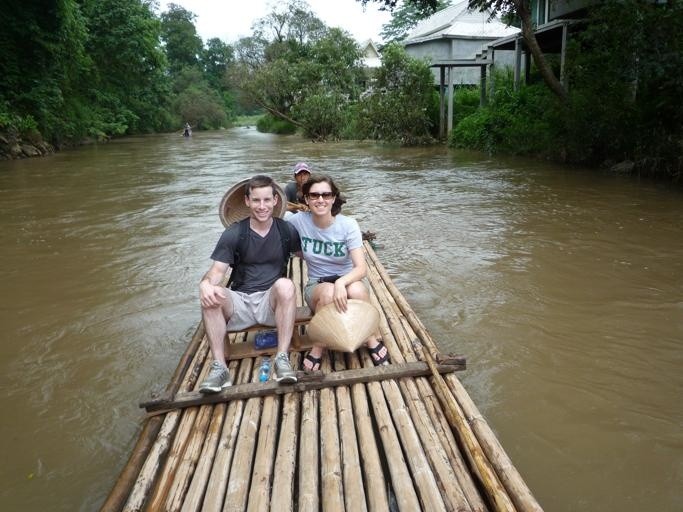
[100,230,544,512]
[185,129,192,137]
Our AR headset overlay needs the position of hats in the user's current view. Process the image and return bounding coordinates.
[219,179,285,229]
[293,162,311,175]
[308,299,380,353]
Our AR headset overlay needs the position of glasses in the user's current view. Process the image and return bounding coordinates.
[306,191,334,201]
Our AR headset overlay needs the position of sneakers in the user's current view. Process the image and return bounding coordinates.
[198,361,232,393]
[273,354,298,384]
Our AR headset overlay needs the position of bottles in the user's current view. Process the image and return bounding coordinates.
[257,357,272,383]
[254,331,278,350]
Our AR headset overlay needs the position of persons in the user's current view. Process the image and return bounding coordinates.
[199,175,303,392]
[283,163,311,220]
[286,174,390,371]
[185,123,189,137]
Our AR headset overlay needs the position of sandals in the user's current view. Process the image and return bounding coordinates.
[369,341,388,364]
[304,352,322,372]
